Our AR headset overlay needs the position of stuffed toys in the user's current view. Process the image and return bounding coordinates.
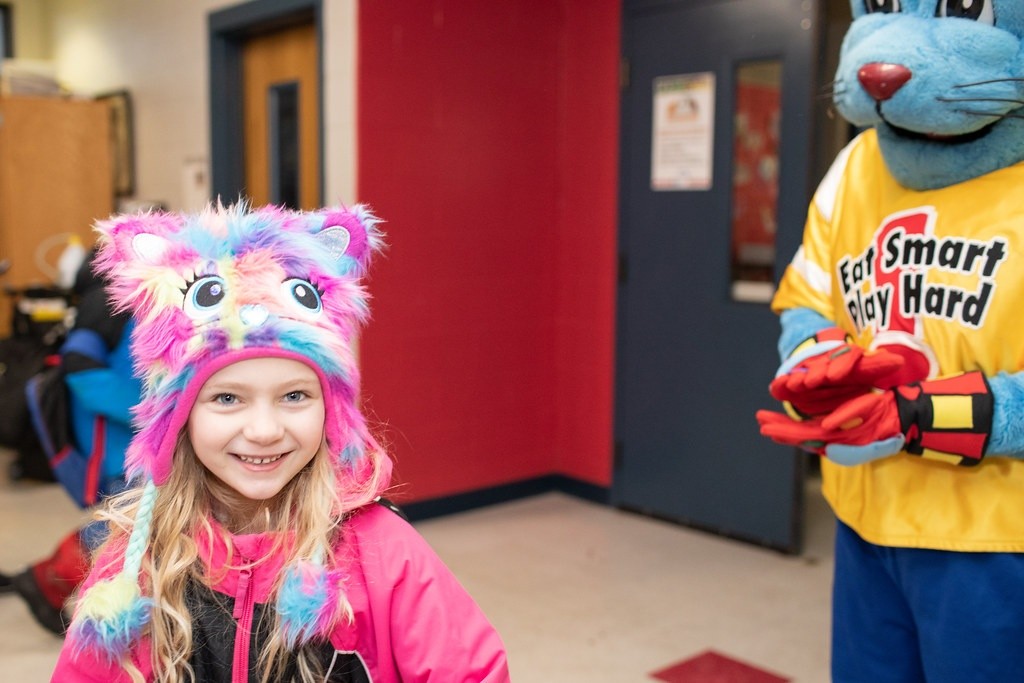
[749,2,1023,683]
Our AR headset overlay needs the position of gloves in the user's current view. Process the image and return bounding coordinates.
[755,370,993,467]
[767,327,906,417]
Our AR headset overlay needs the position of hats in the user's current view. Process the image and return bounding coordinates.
[66,192,394,666]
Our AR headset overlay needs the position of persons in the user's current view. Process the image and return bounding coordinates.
[48,200,510,683]
